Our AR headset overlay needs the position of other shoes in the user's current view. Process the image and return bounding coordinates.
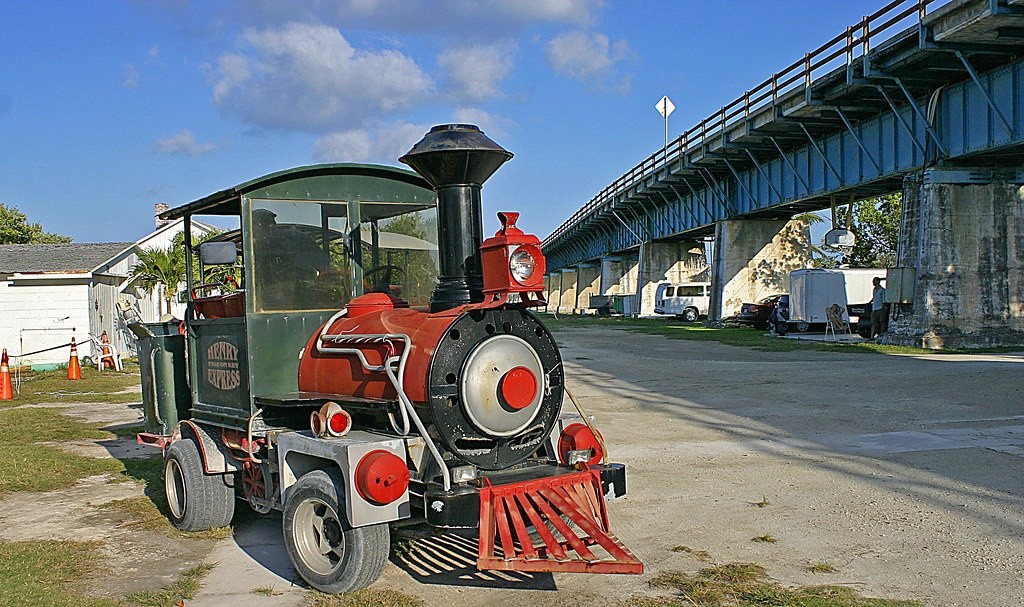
[865,338,875,343]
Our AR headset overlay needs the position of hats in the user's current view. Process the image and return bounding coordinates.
[252,209,277,237]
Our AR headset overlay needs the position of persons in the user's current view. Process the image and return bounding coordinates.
[827,304,846,330]
[866,277,885,342]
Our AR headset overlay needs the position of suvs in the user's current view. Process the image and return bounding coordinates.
[652,281,713,323]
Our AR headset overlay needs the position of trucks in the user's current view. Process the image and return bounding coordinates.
[786,265,892,338]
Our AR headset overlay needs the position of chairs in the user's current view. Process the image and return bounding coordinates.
[87,332,124,372]
[192,281,246,319]
[824,313,854,342]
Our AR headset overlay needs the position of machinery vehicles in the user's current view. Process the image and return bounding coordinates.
[125,121,645,595]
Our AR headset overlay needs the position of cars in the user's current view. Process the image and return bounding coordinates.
[737,293,826,333]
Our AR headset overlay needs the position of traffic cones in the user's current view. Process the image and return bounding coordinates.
[99,331,115,369]
[0,347,14,401]
[66,336,83,382]
